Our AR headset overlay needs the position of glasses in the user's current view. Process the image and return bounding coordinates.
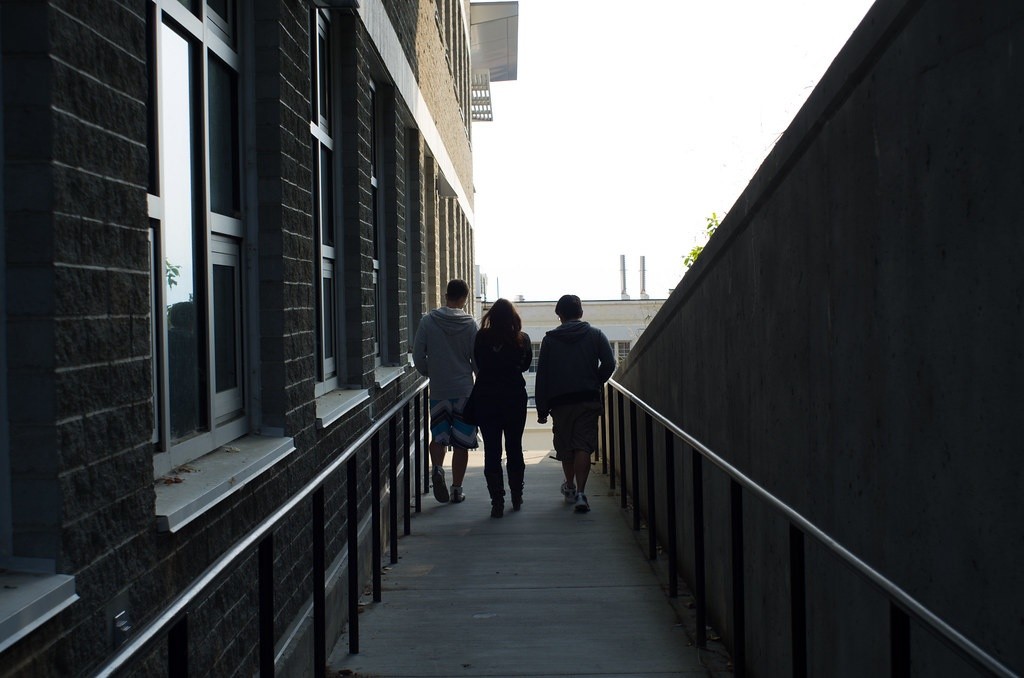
[558,317,561,321]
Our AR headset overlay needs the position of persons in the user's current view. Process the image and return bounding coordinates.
[473,299,533,519]
[412,279,479,505]
[535,295,617,514]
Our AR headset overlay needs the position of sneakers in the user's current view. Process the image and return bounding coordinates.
[491,504,505,518]
[561,482,576,502]
[432,465,449,503]
[575,492,590,512]
[513,503,521,510]
[450,485,466,503]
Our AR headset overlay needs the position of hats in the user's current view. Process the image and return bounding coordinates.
[555,295,583,318]
